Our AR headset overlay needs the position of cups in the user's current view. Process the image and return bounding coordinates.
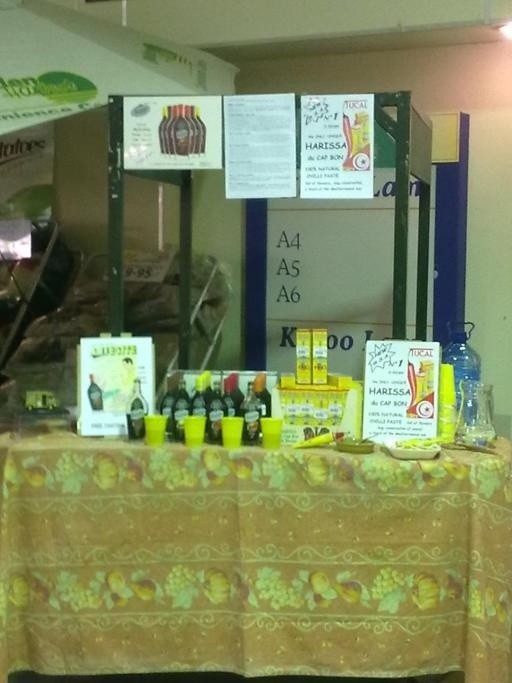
[260,417,284,450]
[144,415,167,447]
[221,417,245,448]
[183,415,207,448]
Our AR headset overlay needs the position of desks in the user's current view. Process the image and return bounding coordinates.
[1,423,511,682]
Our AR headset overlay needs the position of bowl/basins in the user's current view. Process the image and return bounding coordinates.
[336,437,374,454]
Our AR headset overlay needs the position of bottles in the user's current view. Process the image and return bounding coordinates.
[157,104,207,156]
[437,363,457,444]
[87,374,104,411]
[441,330,481,425]
[125,379,149,440]
[160,370,271,445]
[457,380,497,450]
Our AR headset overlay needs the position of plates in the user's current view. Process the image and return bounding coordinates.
[384,439,441,459]
